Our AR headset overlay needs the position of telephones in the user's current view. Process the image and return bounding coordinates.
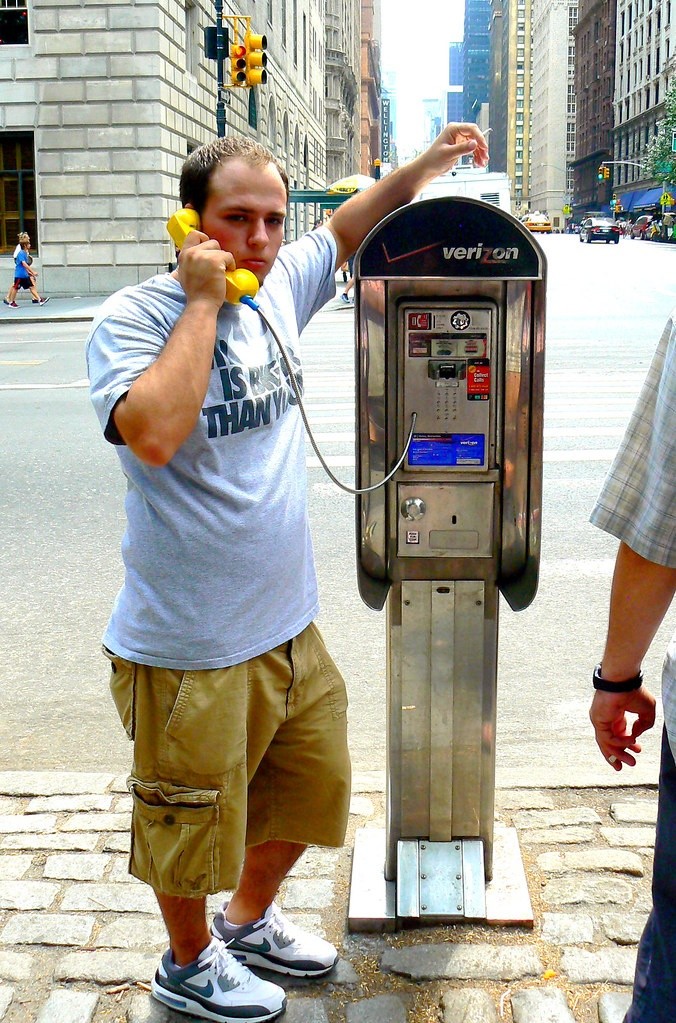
[166,209,259,305]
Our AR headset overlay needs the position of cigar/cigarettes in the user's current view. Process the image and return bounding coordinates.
[609,734,636,763]
[483,128,493,137]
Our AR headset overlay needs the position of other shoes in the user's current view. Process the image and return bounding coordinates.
[3,299,9,305]
[341,293,350,303]
[9,301,20,309]
[351,297,354,303]
[32,298,39,303]
[38,297,50,306]
[344,276,347,282]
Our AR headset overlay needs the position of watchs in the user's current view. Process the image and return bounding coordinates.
[593,663,643,693]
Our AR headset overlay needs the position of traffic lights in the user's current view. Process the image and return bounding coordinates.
[598,168,603,179]
[230,45,247,85]
[245,30,267,87]
[604,168,610,179]
[613,194,616,200]
[616,206,619,213]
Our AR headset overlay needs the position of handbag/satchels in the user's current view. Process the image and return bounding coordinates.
[341,261,350,272]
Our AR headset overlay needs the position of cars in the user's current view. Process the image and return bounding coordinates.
[523,211,552,234]
[579,217,619,244]
[630,215,663,240]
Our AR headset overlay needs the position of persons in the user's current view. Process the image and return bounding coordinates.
[554,218,585,234]
[589,314,676,1023]
[4,232,50,308]
[311,219,356,303]
[617,219,632,239]
[84,122,490,1023]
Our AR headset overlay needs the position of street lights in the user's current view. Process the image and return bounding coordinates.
[541,163,571,219]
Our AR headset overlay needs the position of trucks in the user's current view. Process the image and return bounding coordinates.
[411,173,511,214]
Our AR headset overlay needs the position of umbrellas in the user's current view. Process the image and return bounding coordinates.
[324,174,378,194]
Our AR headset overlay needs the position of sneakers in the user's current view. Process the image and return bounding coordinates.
[209,900,339,978]
[151,935,287,1023]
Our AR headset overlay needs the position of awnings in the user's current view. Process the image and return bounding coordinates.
[602,185,676,218]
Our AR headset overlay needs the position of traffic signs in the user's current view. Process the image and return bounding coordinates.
[609,206,616,211]
